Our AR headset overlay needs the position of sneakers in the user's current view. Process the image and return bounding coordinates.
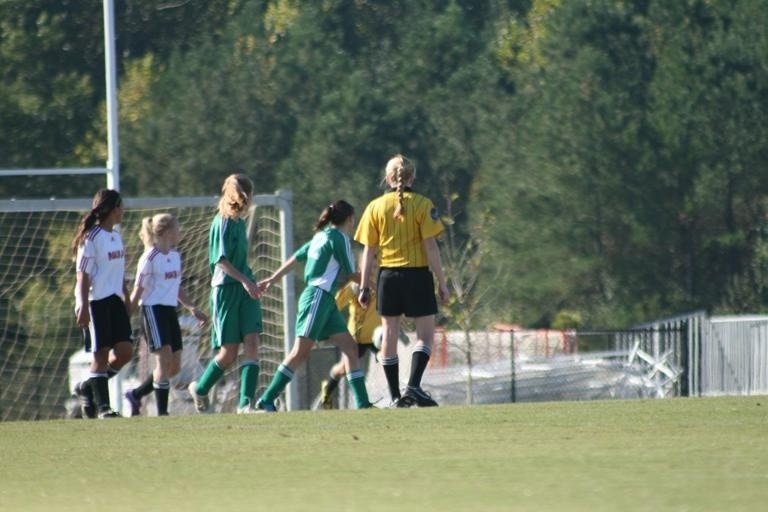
[322,381,332,410]
[391,398,408,408]
[367,404,377,409]
[255,398,276,411]
[98,405,120,418]
[125,390,141,416]
[402,385,438,407]
[188,382,209,413]
[74,381,97,419]
[237,402,266,414]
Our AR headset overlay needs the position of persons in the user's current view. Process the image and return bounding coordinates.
[69,188,134,418]
[127,212,208,417]
[187,173,270,415]
[255,200,381,412]
[311,250,381,411]
[352,154,451,409]
